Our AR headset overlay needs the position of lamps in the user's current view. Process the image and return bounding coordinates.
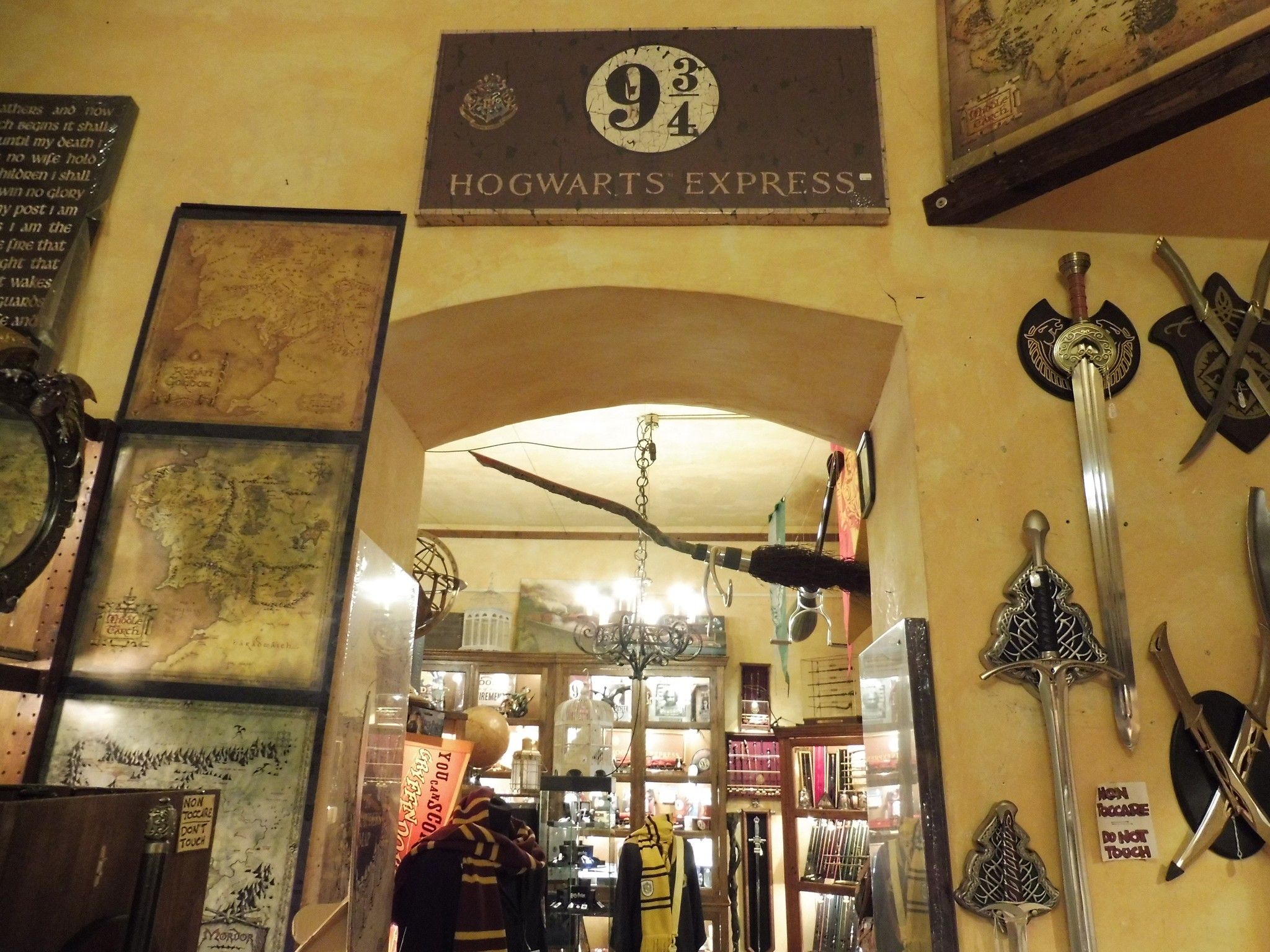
[569,410,711,682]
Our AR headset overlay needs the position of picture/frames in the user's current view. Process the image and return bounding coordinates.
[855,430,876,520]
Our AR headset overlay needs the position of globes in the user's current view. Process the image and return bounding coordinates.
[455,705,512,796]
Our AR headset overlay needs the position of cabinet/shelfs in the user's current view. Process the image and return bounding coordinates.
[553,647,731,951]
[418,651,553,844]
[536,773,620,952]
[772,721,875,952]
